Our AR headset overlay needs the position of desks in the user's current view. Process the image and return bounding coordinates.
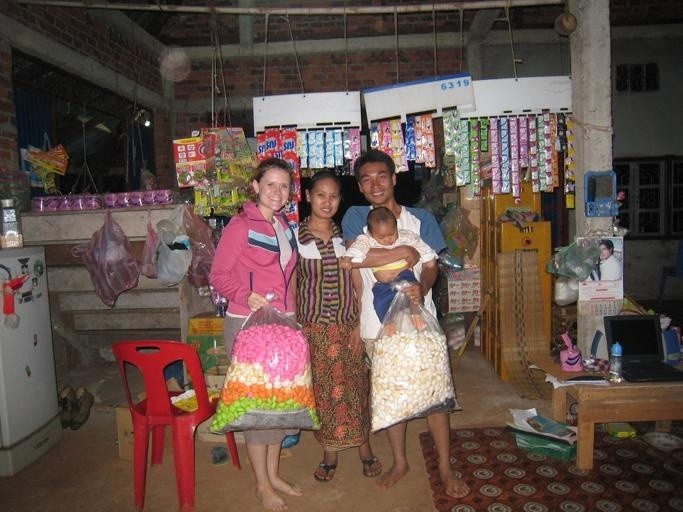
[551,366,683,470]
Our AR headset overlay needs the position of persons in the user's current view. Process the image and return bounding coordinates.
[596,239,622,281]
[289,171,385,484]
[208,157,303,512]
[341,148,470,499]
[339,206,439,338]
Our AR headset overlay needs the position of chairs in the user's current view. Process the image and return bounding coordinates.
[111,340,241,512]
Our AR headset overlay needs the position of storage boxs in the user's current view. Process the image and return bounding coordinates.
[116,377,184,463]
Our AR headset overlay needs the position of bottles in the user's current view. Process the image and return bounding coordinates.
[0,196,27,249]
[607,343,622,379]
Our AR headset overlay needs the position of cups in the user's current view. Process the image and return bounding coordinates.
[31,188,175,213]
[215,305,227,318]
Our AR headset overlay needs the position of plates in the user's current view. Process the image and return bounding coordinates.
[642,432,682,452]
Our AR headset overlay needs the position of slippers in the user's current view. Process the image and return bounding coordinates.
[362,454,381,478]
[314,457,335,481]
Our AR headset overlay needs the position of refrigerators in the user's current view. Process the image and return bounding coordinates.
[0,244,64,480]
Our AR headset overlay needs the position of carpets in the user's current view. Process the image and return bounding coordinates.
[420,421,683,512]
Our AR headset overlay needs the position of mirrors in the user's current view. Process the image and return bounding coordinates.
[585,171,619,217]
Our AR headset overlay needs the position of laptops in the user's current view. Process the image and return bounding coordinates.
[602,314,682,382]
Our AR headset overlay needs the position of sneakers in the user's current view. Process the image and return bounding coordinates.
[58,387,93,430]
